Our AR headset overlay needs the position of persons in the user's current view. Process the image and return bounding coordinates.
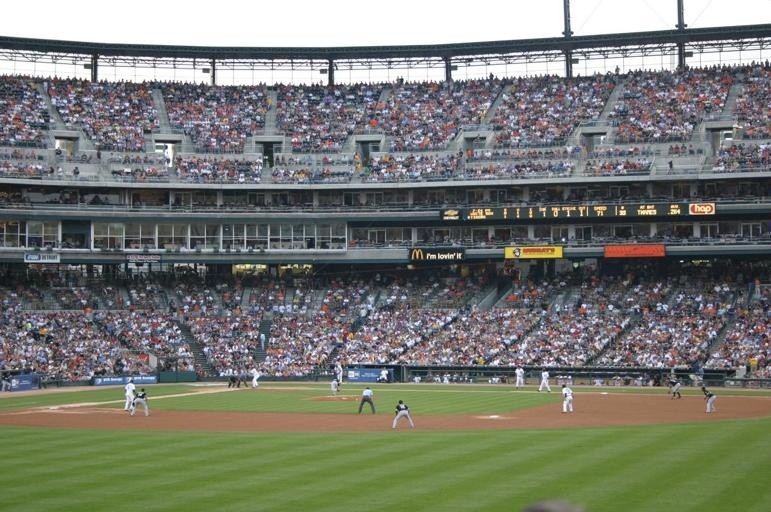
[130,387,150,417]
[539,368,553,391]
[2,56,770,390]
[559,383,575,414]
[358,383,377,413]
[665,374,683,402]
[700,385,718,413]
[391,398,417,430]
[329,378,339,395]
[121,379,137,410]
